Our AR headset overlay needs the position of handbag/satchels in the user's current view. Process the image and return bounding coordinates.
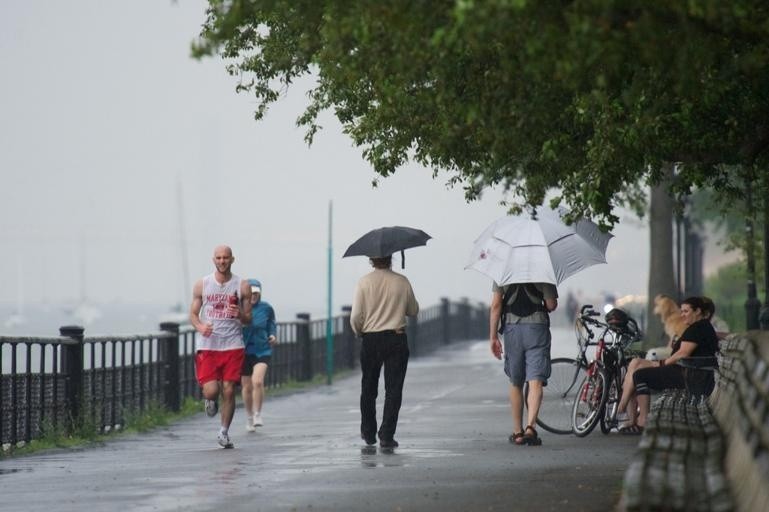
[645,365,680,390]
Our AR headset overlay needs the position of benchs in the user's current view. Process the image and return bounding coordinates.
[618,331,768,509]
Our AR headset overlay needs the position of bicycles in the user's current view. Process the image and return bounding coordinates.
[570,307,626,438]
[519,300,649,436]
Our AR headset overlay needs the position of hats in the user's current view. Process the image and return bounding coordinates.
[250,285,260,295]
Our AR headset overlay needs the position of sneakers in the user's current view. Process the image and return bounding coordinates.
[610,406,642,435]
[252,414,263,427]
[203,399,218,417]
[216,432,233,448]
[246,421,255,432]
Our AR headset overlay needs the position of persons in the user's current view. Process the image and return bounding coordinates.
[645,292,679,347]
[489,279,559,447]
[349,253,419,448]
[240,279,277,432]
[189,245,253,448]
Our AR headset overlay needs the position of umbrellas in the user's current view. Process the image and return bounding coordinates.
[342,225,433,261]
[463,202,616,287]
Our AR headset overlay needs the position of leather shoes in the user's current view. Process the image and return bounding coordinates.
[380,438,398,448]
[360,434,376,445]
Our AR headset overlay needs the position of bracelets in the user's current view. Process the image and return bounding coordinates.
[658,359,666,366]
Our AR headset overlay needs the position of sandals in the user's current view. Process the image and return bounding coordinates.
[508,430,524,445]
[524,426,541,446]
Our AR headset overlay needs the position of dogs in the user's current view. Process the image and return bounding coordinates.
[651,293,691,352]
